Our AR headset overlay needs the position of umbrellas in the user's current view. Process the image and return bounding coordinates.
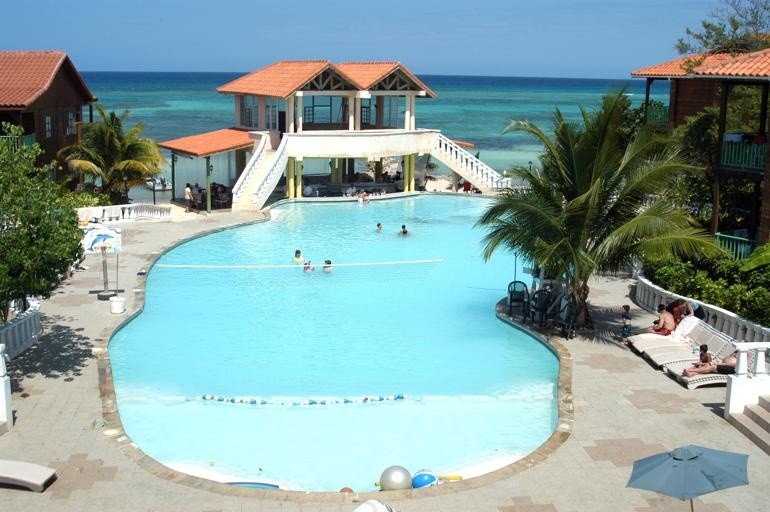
[626,445,750,512]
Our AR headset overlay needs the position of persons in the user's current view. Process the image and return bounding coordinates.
[639,298,707,336]
[682,338,752,377]
[382,170,403,184]
[302,183,313,197]
[687,345,711,369]
[294,250,332,273]
[622,305,632,338]
[400,225,408,234]
[376,223,382,232]
[184,182,232,213]
[402,230,407,236]
[344,165,375,206]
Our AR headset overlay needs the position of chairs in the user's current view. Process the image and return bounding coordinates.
[625,314,759,391]
[508,280,580,341]
